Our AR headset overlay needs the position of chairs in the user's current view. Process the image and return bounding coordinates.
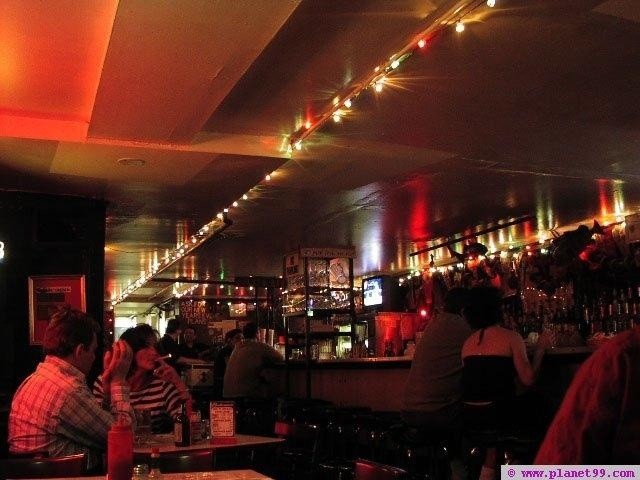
[0,450,88,480]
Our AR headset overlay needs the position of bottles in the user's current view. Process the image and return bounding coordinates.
[149,448,163,480]
[404,341,415,357]
[385,343,393,357]
[542,323,554,351]
[583,276,640,338]
[508,270,517,288]
[107,414,134,480]
[502,304,582,339]
[191,409,201,442]
[173,398,191,447]
[131,463,148,480]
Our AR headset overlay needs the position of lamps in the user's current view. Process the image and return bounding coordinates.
[402,212,535,270]
[149,274,282,308]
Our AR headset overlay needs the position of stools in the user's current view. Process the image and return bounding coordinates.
[221,395,547,480]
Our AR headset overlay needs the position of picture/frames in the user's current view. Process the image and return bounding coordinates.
[28,274,86,346]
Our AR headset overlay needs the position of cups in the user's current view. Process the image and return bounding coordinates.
[311,344,319,360]
[528,332,538,342]
[330,264,347,285]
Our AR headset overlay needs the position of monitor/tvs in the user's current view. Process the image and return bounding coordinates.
[362,276,399,310]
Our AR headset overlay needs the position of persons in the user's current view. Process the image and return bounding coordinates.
[532,325,640,465]
[400,283,479,479]
[458,283,556,479]
[5,301,285,467]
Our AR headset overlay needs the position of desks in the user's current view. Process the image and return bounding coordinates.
[37,467,274,480]
[127,435,285,472]
